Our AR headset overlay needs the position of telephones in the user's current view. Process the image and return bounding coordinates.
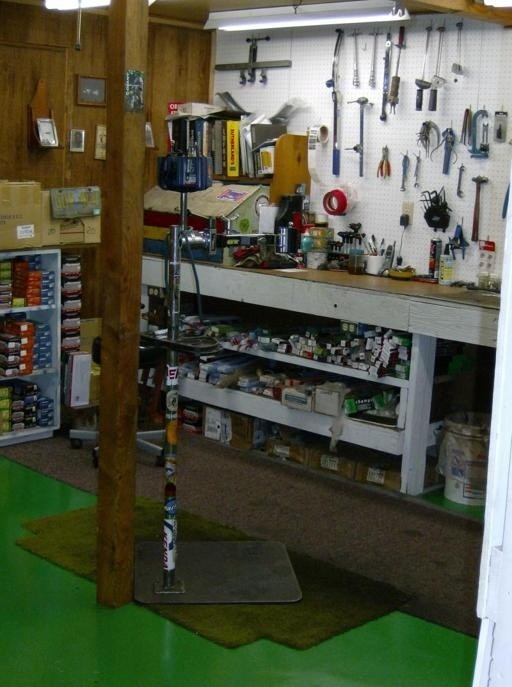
[378,241,396,277]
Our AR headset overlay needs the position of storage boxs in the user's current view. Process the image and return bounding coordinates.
[1,177,41,253]
[40,183,103,246]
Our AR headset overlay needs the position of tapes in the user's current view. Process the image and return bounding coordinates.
[323,189,347,215]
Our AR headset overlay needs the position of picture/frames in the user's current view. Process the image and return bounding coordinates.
[76,72,107,106]
[36,117,59,147]
[93,124,108,160]
[68,127,86,153]
[145,119,156,148]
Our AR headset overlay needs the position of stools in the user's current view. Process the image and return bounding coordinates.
[66,337,168,466]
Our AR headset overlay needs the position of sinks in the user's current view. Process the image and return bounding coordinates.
[419,288,500,310]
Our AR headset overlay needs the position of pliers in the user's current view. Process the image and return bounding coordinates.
[377,144,391,179]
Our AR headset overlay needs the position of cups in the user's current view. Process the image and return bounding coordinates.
[366,256,383,275]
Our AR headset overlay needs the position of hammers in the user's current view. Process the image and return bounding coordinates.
[471,176,488,241]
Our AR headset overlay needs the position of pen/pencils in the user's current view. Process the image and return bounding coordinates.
[358,230,385,256]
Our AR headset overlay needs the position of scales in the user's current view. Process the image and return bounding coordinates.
[133,153,303,605]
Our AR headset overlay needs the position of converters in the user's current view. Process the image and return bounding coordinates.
[400,214,409,225]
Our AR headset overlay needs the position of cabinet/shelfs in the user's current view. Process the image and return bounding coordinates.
[212,133,317,209]
[0,243,65,449]
[135,248,505,502]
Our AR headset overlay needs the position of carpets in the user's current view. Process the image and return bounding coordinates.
[14,492,418,656]
[0,426,481,639]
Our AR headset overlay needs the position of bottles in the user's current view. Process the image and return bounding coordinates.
[476,240,497,291]
[350,248,365,274]
[278,228,295,252]
[300,229,313,253]
[437,254,455,286]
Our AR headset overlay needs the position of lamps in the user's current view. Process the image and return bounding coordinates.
[201,0,413,36]
